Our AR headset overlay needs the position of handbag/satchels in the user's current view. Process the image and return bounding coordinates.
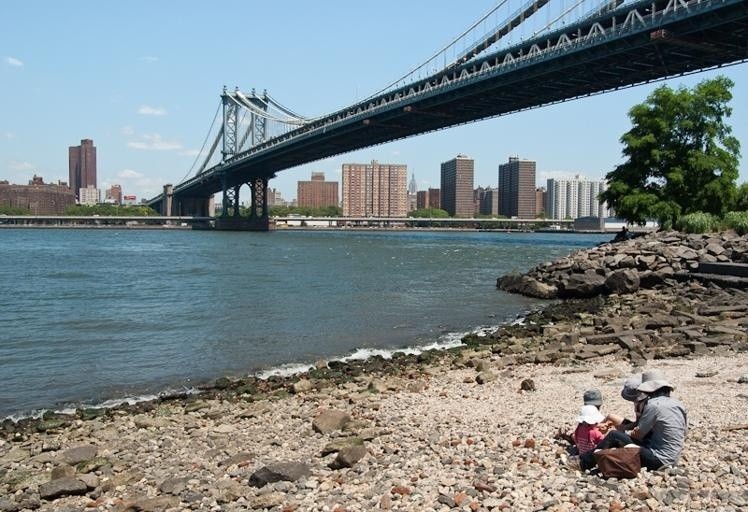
[594,447,641,479]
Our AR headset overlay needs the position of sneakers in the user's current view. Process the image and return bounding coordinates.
[560,453,581,471]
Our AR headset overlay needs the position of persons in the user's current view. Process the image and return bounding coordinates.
[601,379,649,433]
[583,388,613,435]
[574,405,605,456]
[560,368,688,471]
[615,226,632,242]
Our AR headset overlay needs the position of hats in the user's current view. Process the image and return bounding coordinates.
[621,378,648,402]
[636,369,676,392]
[574,405,605,424]
[584,389,603,405]
